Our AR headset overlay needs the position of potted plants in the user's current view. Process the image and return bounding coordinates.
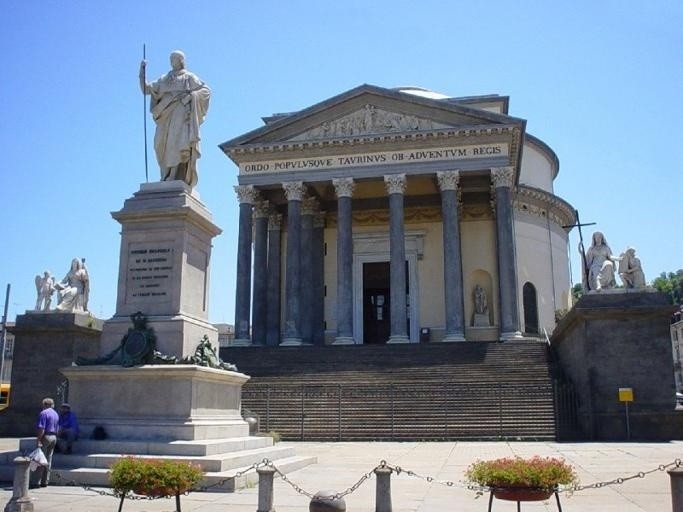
[105,452,201,497]
[461,452,579,503]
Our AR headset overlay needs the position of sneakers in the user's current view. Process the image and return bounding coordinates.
[29,484,47,489]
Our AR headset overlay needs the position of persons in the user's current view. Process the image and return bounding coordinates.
[617,248,645,289]
[138,50,211,188]
[473,282,487,315]
[37,269,55,312]
[577,231,623,292]
[28,395,59,487]
[54,402,79,455]
[301,103,432,140]
[52,258,88,313]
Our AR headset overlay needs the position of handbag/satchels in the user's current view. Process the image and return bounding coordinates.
[93,427,104,439]
